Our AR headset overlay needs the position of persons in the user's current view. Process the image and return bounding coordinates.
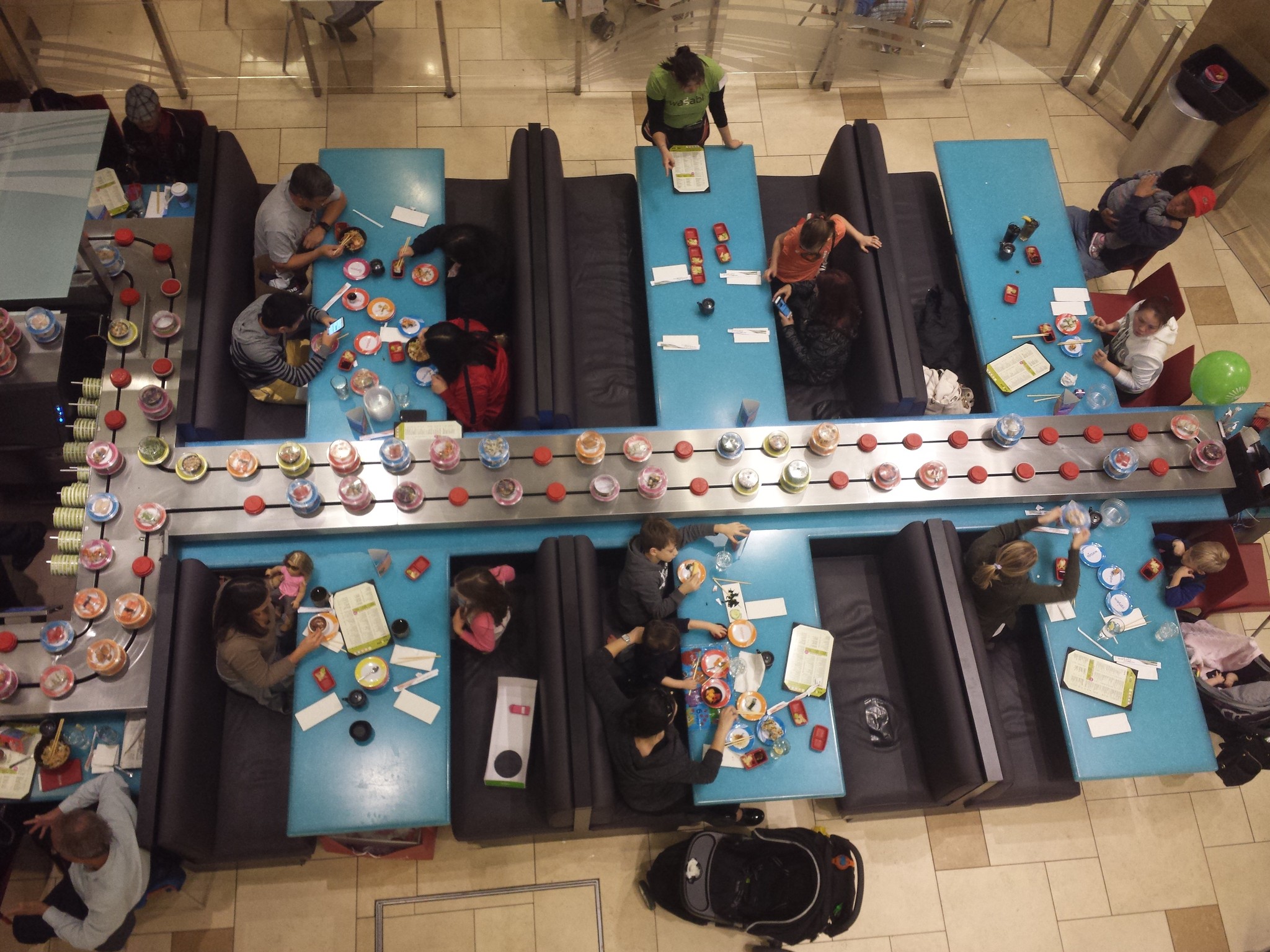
[418,316,510,432]
[1204,669,1245,692]
[0,520,47,611]
[761,213,883,288]
[616,515,751,628]
[120,83,202,184]
[322,0,384,44]
[771,268,862,386]
[397,222,504,300]
[1087,296,1179,403]
[641,45,744,178]
[229,290,341,406]
[582,627,765,827]
[211,574,325,716]
[264,550,315,638]
[1152,532,1230,608]
[965,505,1092,643]
[606,617,728,692]
[254,163,348,304]
[450,564,516,655]
[1064,177,1217,282]
[2,771,152,952]
[1088,164,1198,260]
[1252,405,1270,428]
[29,87,125,186]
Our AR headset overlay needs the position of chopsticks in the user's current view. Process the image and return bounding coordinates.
[157,184,160,213]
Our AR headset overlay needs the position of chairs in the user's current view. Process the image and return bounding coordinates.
[1089,262,1186,348]
[1159,520,1270,638]
[1115,344,1195,408]
[280,1,376,89]
[797,0,882,85]
[1116,250,1159,295]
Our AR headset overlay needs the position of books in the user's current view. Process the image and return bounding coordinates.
[39,758,83,792]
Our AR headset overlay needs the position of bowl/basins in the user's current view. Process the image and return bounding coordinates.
[685,219,733,315]
[307,588,411,742]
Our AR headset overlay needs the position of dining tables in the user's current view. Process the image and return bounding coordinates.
[1016,516,1219,781]
[672,528,847,807]
[933,138,1122,415]
[306,147,447,436]
[0,708,147,804]
[287,548,450,838]
[635,145,790,428]
[85,183,197,220]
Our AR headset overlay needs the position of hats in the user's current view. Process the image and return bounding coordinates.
[1188,185,1216,218]
[124,83,159,125]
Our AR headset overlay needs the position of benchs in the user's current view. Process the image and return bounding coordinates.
[811,518,1083,823]
[450,534,700,848]
[156,558,316,873]
[176,124,309,443]
[444,121,658,432]
[756,119,987,423]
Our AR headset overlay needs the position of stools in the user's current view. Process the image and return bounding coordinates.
[135,852,187,909]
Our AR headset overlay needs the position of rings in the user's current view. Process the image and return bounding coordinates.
[731,709,735,715]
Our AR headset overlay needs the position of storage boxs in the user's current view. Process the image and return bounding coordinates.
[1175,44,1270,126]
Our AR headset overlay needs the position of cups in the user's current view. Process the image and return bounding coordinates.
[170,182,192,208]
[1250,403,1270,432]
[124,183,146,220]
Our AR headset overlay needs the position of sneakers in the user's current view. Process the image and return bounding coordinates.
[1089,232,1105,259]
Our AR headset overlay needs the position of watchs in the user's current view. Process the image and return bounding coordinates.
[318,222,331,232]
[621,633,630,646]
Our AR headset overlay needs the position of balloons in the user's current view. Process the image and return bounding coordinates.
[1189,350,1252,406]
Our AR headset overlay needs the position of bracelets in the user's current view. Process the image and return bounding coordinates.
[270,578,278,589]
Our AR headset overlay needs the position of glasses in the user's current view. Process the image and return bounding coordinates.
[313,198,328,207]
[799,244,824,255]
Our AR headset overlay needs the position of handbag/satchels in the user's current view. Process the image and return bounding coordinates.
[920,282,961,349]
[812,398,853,421]
[923,364,975,415]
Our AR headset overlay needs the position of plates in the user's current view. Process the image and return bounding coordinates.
[1000,211,1116,415]
[0,233,182,779]
[310,219,441,419]
[1198,64,1229,92]
[678,524,831,769]
[184,413,1227,517]
[1056,517,1182,663]
